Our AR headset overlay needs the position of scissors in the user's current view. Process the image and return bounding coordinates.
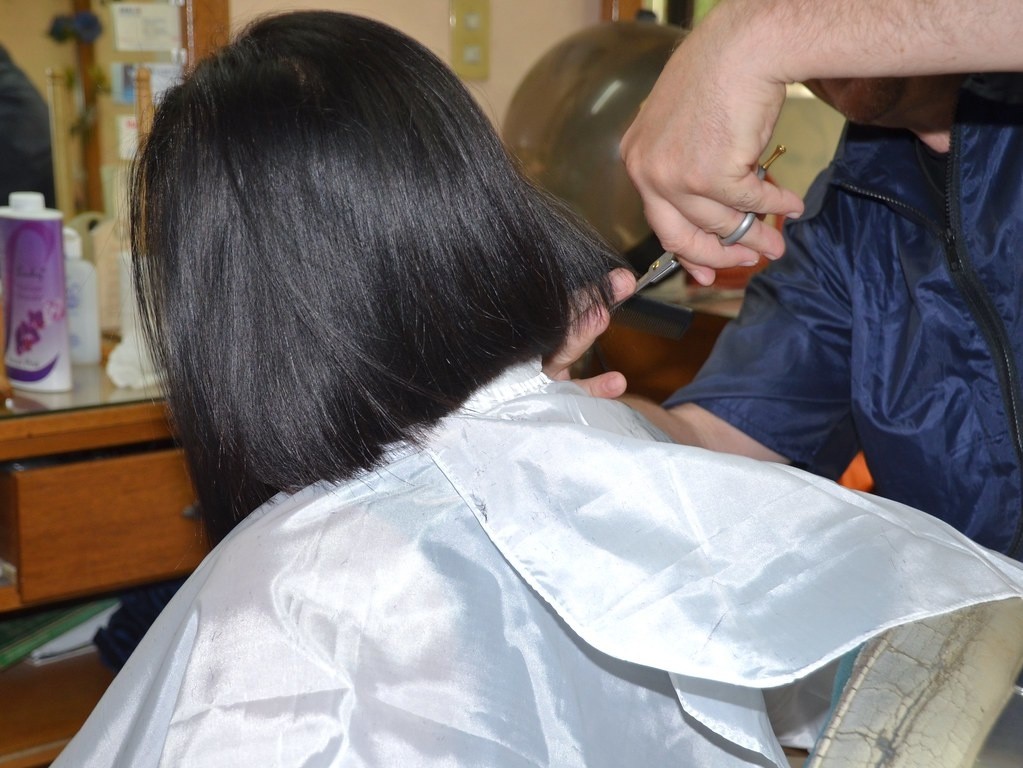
[612,165,770,310]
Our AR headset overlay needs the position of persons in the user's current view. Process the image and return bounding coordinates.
[49,7,790,768]
[541,0,1023,685]
[1,43,57,214]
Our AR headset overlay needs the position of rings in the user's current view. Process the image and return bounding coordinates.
[719,212,758,248]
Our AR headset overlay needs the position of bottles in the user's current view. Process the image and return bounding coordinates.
[1,192,77,395]
[62,225,102,369]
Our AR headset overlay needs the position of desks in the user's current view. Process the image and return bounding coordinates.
[0,330,206,767]
